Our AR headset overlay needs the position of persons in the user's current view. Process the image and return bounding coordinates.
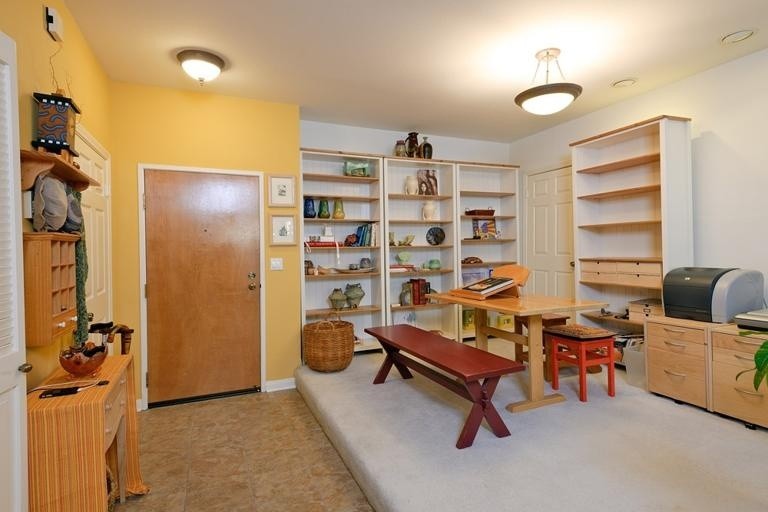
[419,182,431,195]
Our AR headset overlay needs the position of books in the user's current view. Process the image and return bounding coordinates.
[462,277,514,295]
[401,278,431,305]
[390,268,414,272]
[352,223,379,247]
[390,265,414,268]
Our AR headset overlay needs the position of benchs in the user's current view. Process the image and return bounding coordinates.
[363,324,527,449]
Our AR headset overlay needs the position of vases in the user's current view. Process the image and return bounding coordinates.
[423,200,435,220]
[333,199,345,220]
[304,199,316,217]
[396,140,406,156]
[419,136,432,159]
[405,132,420,157]
[318,199,330,218]
[405,175,419,195]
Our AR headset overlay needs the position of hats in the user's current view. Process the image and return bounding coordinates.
[33,170,82,232]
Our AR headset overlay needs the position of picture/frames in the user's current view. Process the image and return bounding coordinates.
[268,212,298,246]
[266,172,296,208]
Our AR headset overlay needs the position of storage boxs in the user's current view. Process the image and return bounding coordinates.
[623,345,645,388]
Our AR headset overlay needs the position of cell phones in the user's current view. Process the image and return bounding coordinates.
[38,386,79,398]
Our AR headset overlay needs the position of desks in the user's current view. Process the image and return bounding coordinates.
[424,292,610,414]
[27,354,153,512]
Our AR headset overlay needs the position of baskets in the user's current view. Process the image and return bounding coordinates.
[303,311,354,372]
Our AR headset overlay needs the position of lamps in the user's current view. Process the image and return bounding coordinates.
[177,49,226,87]
[514,46,583,116]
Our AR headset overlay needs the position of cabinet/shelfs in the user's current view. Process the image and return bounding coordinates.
[301,147,386,366]
[707,325,768,429]
[457,161,521,343]
[644,316,735,412]
[383,155,459,343]
[22,230,78,348]
[569,114,694,366]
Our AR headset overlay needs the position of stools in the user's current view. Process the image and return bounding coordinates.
[543,323,618,401]
[514,313,571,381]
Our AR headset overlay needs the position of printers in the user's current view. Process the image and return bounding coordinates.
[663,266,764,324]
